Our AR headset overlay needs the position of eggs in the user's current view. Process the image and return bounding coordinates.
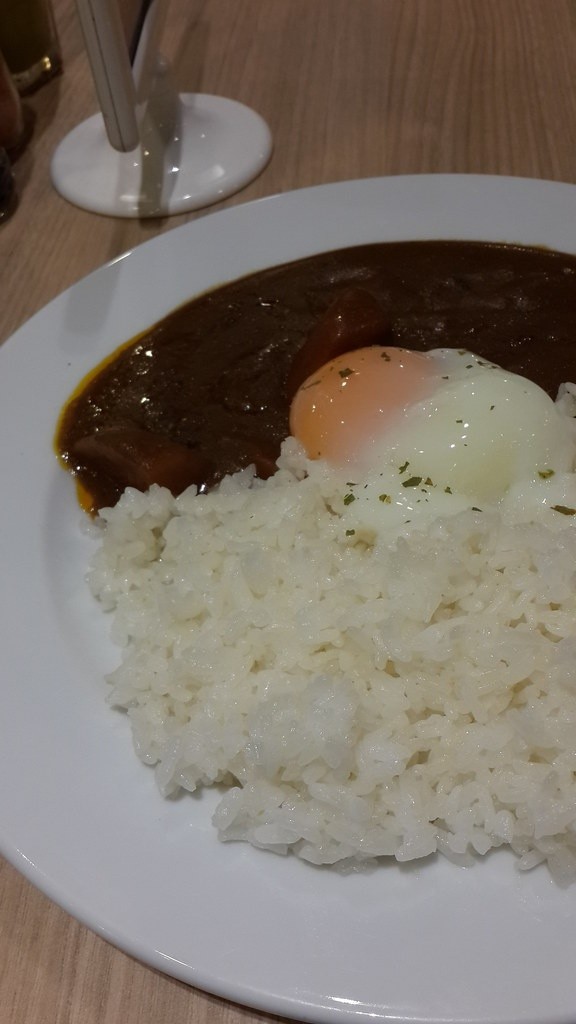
[286,344,576,497]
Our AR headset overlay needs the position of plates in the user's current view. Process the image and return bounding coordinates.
[0,175,576,1024]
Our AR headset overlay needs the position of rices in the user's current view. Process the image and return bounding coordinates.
[73,435,576,892]
